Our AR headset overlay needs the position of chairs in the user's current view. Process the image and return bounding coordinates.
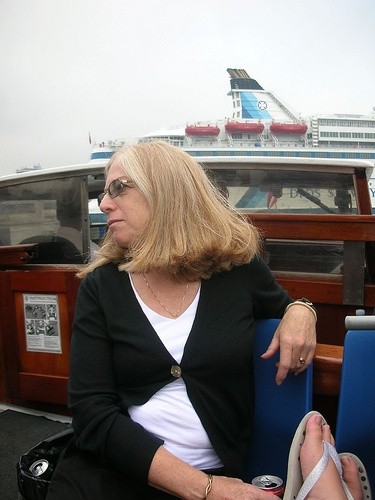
[248,317,312,486]
[335,316,375,500]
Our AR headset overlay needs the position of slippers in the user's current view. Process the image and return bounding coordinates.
[282,409,342,500]
[337,453,372,500]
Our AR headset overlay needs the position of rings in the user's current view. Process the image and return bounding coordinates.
[299,358,305,365]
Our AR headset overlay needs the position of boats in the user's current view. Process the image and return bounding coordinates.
[1,67,375,278]
[224,120,265,134]
[185,124,220,137]
[269,122,307,135]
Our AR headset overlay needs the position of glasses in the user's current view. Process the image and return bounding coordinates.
[98,178,133,207]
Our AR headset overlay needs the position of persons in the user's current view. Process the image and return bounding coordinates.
[48,140,313,500]
[282,408,373,500]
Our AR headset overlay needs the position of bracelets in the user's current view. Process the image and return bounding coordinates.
[284,301,317,321]
[201,474,214,500]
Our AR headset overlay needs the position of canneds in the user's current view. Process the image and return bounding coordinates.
[252,475,285,500]
[28,458,55,481]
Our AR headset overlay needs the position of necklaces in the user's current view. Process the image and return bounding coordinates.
[142,272,189,318]
